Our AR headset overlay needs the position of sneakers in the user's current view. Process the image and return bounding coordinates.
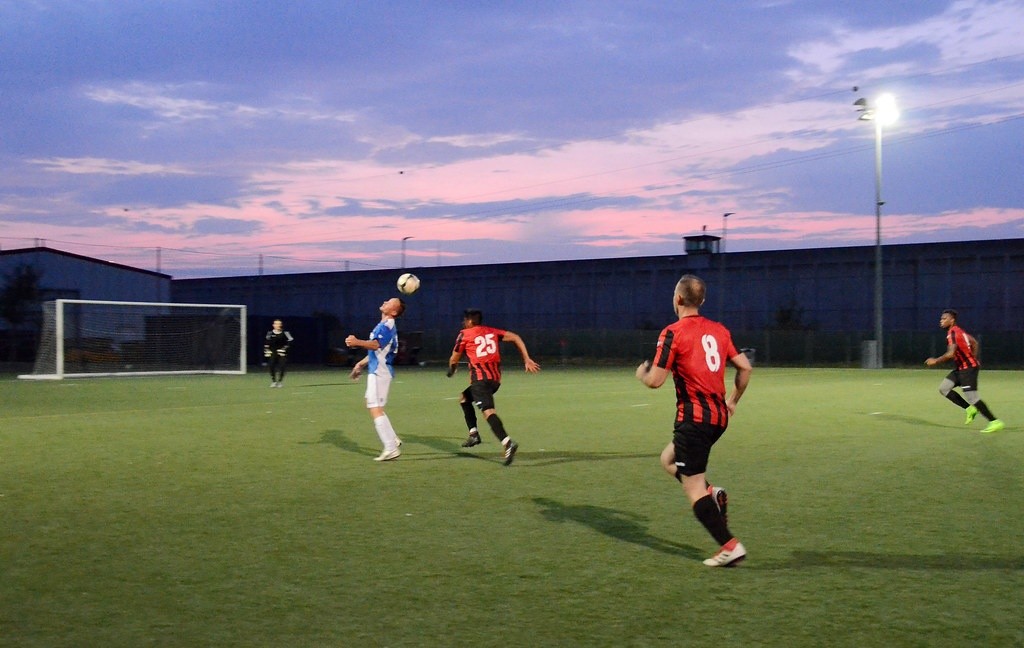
[373,447,401,461]
[965,404,978,424]
[980,418,1006,433]
[708,485,728,527]
[462,435,481,448]
[502,442,518,466]
[394,437,401,447]
[703,538,747,567]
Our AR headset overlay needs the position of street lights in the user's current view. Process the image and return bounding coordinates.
[853,94,898,368]
[718,213,735,251]
[400,235,414,267]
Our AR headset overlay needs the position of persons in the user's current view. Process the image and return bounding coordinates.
[449,309,541,466]
[635,275,752,566]
[345,298,406,462]
[264,319,294,387]
[923,310,1005,433]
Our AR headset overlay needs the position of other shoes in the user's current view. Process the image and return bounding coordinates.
[269,381,283,388]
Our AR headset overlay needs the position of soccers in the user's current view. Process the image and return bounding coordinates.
[397,273,421,294]
